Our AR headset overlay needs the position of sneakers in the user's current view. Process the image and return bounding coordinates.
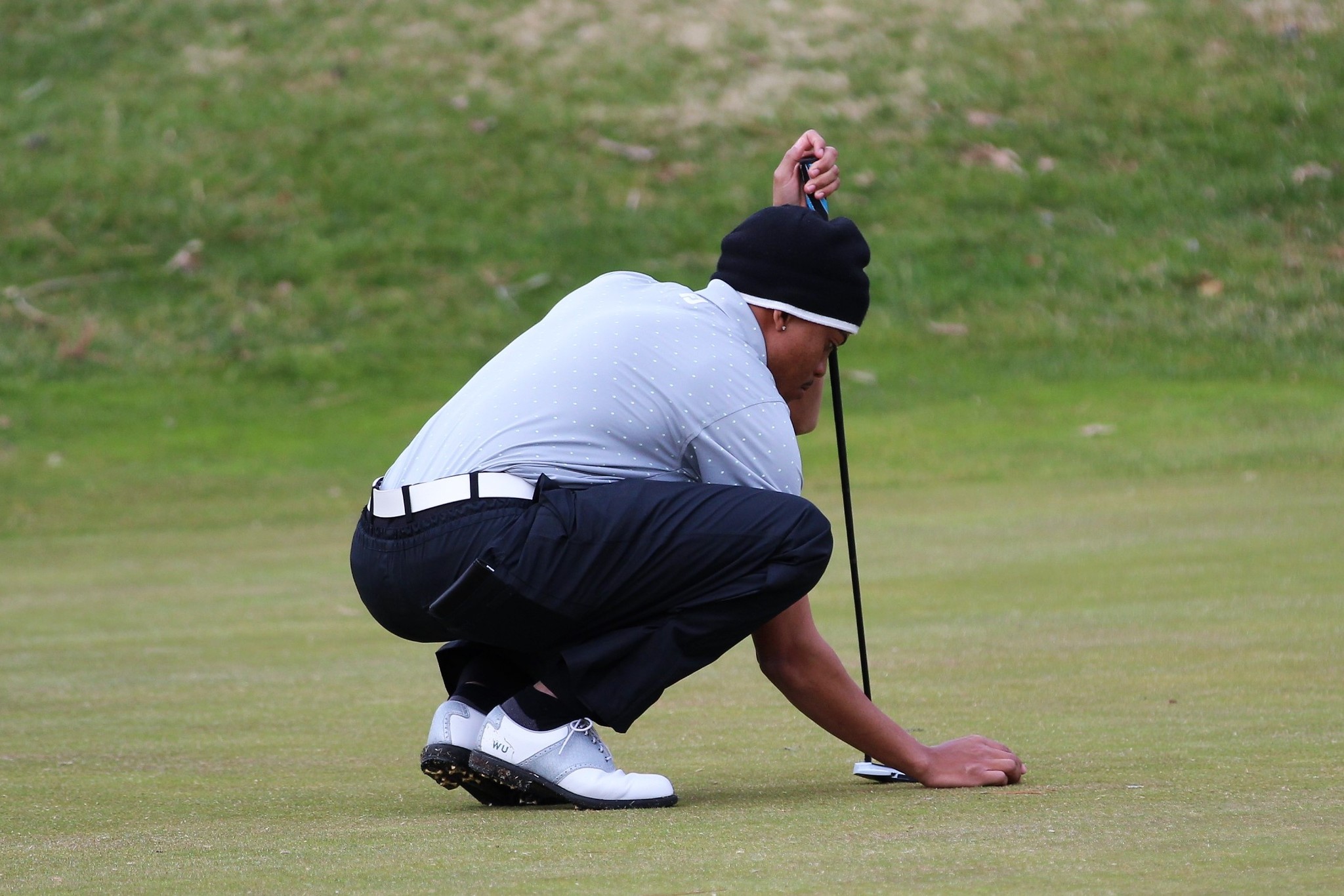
[421,700,538,806]
[469,704,678,809]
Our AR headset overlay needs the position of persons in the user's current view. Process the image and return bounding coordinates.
[348,128,1028,808]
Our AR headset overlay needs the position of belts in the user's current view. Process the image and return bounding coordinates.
[367,472,536,518]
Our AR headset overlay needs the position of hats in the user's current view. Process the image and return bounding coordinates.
[710,204,870,335]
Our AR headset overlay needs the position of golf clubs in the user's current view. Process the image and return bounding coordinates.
[799,153,912,782]
